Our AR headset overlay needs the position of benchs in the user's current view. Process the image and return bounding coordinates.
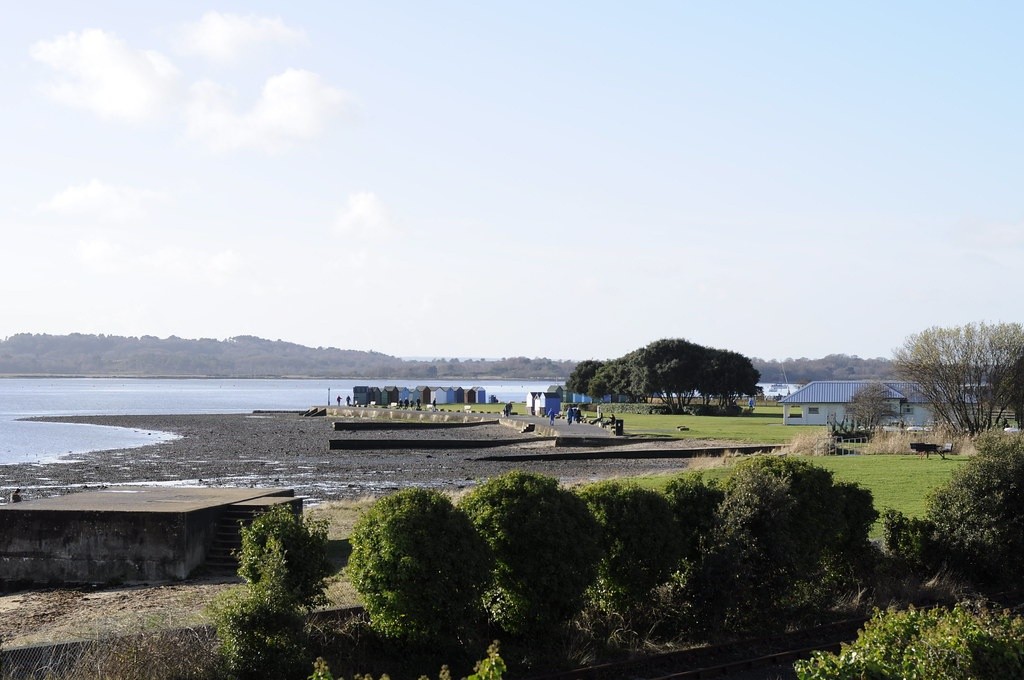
[580,414,587,423]
[425,404,433,411]
[388,403,397,408]
[367,401,376,407]
[599,422,616,430]
[942,443,953,459]
[588,418,599,425]
[463,405,471,413]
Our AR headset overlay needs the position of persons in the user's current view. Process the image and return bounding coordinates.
[589,411,616,428]
[503,401,512,418]
[749,397,755,410]
[547,408,556,426]
[399,397,421,409]
[10,488,22,502]
[346,395,351,405]
[432,398,437,411]
[567,406,581,426]
[337,395,342,407]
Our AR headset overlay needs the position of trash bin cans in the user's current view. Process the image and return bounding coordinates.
[615,419,624,435]
